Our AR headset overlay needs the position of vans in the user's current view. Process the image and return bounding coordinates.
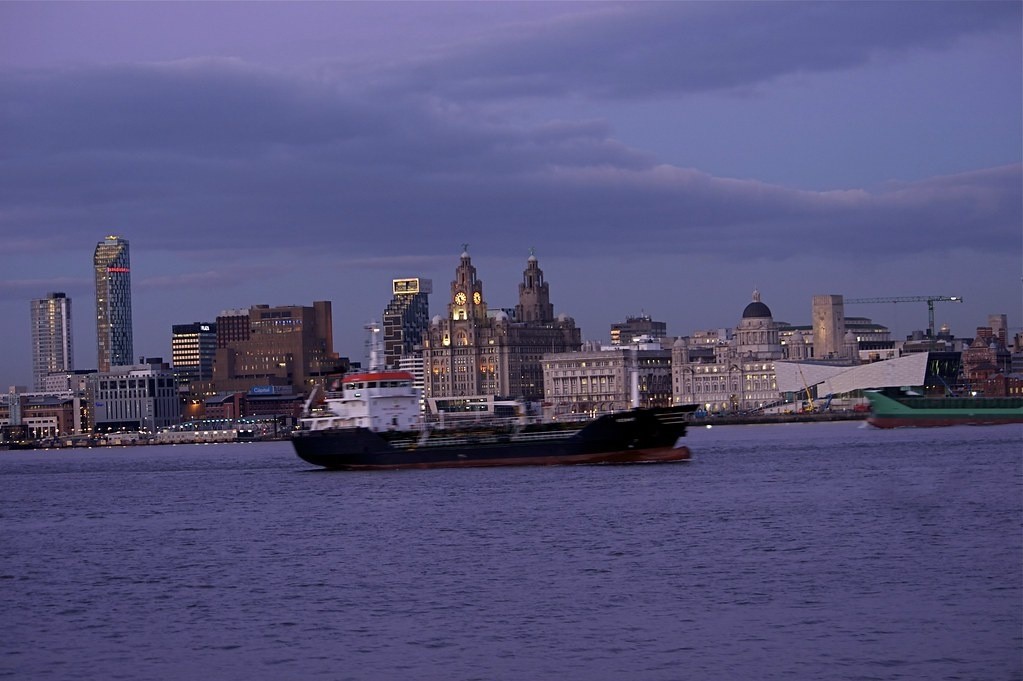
[814,405,822,414]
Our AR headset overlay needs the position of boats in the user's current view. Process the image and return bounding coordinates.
[289,373,702,470]
[863,387,1022,429]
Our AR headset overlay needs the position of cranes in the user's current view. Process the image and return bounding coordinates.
[843,297,963,337]
[794,363,816,414]
